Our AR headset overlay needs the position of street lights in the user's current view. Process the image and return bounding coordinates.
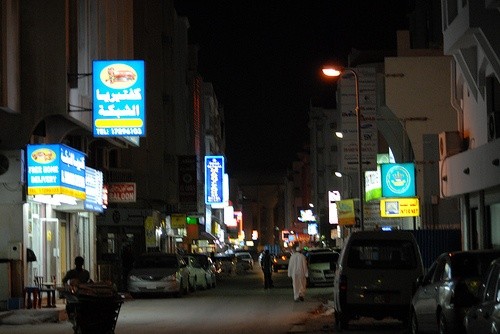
[334,171,352,199]
[322,68,365,231]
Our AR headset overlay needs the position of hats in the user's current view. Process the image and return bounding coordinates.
[296,247,301,251]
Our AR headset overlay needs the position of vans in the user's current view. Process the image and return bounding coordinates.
[331,229,426,331]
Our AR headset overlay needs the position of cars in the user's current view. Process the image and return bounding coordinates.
[271,247,343,288]
[175,247,254,293]
[406,247,500,334]
[126,250,191,300]
[467,257,500,334]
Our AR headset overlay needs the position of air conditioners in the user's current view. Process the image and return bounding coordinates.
[437,131,460,159]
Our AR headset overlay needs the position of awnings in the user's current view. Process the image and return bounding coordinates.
[27,248,37,261]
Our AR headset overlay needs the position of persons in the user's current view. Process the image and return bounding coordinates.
[62,256,90,332]
[287,244,309,301]
[259,249,274,288]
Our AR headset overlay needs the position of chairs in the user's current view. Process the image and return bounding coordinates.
[391,251,401,265]
[33,275,56,308]
[349,250,360,263]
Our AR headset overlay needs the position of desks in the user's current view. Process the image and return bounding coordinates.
[40,281,55,308]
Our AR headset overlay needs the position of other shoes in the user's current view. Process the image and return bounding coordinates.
[299,296,304,301]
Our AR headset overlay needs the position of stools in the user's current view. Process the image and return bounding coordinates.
[25,286,39,309]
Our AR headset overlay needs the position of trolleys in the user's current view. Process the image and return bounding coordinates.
[59,280,123,334]
